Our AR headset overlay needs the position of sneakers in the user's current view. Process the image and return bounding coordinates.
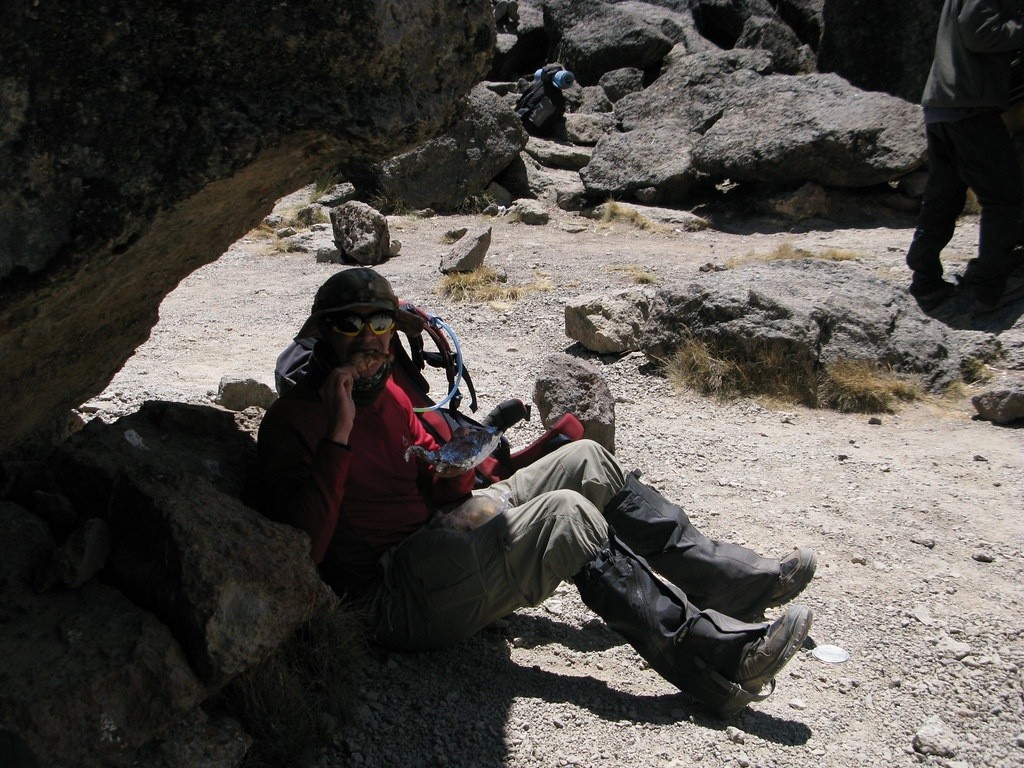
[722,606,811,719]
[755,548,816,623]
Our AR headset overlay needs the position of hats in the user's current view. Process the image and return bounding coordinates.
[296,268,424,339]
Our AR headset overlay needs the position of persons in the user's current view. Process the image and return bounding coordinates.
[266,270,817,718]
[907,0,1024,309]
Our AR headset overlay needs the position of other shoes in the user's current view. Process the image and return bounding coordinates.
[910,280,956,298]
[971,279,1024,312]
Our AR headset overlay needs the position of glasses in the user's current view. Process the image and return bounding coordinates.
[323,310,396,336]
